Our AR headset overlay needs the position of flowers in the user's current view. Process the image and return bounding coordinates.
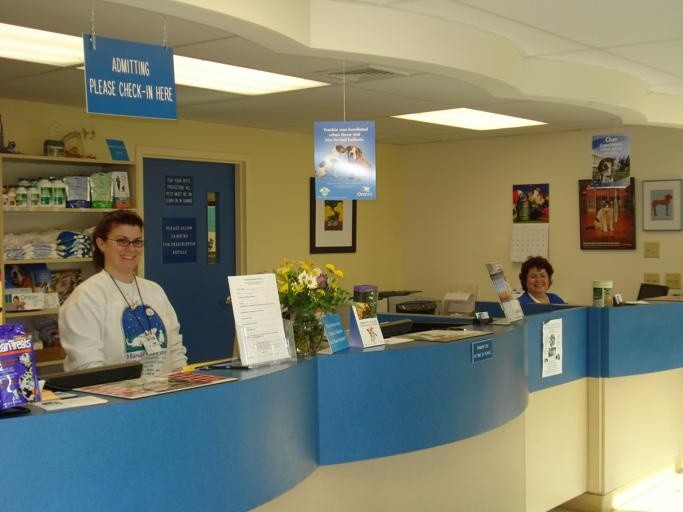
[272,257,351,350]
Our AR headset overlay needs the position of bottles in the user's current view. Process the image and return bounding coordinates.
[16,185,28,209]
[25,178,31,186]
[8,185,17,208]
[51,177,68,208]
[3,186,10,211]
[30,178,38,187]
[44,139,65,157]
[27,185,40,208]
[37,176,43,188]
[17,178,26,186]
[37,176,52,207]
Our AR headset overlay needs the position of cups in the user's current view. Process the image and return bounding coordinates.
[592,279,614,308]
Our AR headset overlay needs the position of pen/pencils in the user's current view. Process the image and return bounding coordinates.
[209,365,253,370]
[448,328,467,331]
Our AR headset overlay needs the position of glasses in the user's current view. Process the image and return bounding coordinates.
[102,237,142,249]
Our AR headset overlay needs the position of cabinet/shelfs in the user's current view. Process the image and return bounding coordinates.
[0,151,138,365]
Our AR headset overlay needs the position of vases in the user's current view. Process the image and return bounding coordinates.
[292,312,323,355]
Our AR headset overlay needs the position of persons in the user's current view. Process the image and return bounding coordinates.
[57,211,188,377]
[518,257,568,304]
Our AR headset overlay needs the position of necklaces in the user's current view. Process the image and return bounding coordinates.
[119,284,137,310]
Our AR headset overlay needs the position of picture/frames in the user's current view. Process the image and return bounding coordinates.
[641,178,683,231]
[311,178,357,254]
